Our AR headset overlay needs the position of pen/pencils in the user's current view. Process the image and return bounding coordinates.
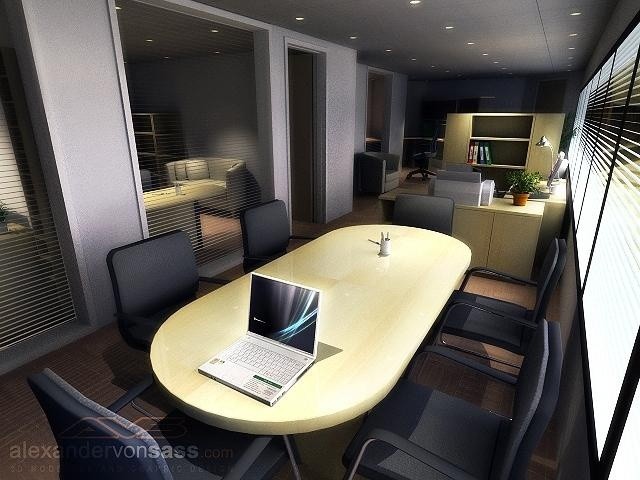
[381,232,389,241]
[368,239,381,245]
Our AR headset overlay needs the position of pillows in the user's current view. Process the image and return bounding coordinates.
[186,160,210,181]
[175,162,187,182]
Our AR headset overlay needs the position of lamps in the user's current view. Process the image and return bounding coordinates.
[535,135,554,176]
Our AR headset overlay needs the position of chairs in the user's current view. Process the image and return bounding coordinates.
[341,319,562,480]
[436,235,568,370]
[236,200,320,273]
[29,367,313,480]
[406,137,438,182]
[106,228,201,414]
[391,194,453,234]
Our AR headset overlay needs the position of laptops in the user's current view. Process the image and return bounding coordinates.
[197,272,323,408]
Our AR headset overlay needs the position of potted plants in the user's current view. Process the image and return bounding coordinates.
[506,170,544,207]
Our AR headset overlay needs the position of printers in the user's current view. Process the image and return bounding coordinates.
[428,168,496,207]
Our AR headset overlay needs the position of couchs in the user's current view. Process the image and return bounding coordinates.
[163,157,247,217]
[357,152,402,195]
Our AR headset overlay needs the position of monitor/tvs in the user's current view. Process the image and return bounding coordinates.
[547,151,565,194]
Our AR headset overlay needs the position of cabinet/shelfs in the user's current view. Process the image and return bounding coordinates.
[486,194,544,282]
[452,195,495,269]
[442,113,565,177]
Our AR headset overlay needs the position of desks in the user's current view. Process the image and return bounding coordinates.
[148,222,472,436]
[502,178,566,240]
